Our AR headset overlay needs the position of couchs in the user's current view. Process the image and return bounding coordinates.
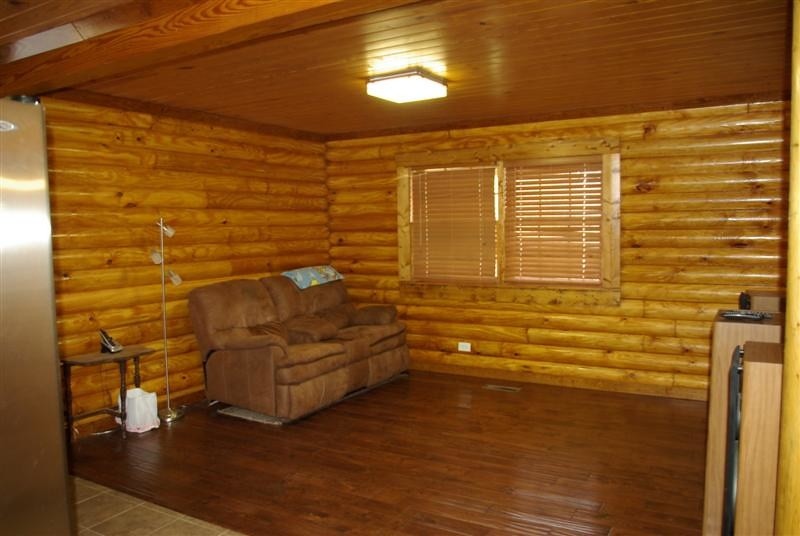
[188,265,410,426]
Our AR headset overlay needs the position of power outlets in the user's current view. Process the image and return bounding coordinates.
[458,341,471,352]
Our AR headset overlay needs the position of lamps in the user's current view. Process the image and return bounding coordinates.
[366,69,447,102]
[151,217,185,423]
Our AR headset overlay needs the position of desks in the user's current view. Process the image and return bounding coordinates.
[61,345,156,452]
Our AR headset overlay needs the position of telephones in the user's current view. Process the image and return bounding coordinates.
[99,330,123,353]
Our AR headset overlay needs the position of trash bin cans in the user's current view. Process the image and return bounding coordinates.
[118,388,157,432]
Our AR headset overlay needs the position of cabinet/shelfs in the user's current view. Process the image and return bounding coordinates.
[704,290,789,536]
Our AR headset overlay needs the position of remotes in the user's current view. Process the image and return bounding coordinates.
[720,309,774,320]
